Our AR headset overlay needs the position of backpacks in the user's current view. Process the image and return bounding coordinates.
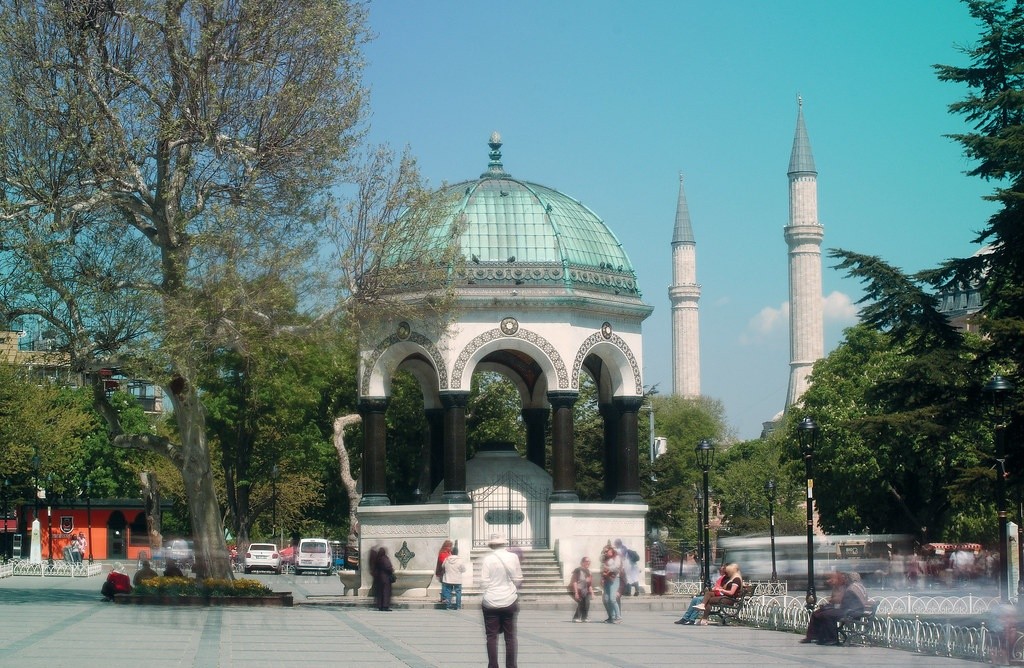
[624,548,640,562]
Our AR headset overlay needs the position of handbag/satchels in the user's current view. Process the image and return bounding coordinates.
[390,574,397,583]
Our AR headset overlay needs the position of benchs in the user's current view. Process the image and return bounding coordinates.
[692,589,747,626]
[821,600,880,647]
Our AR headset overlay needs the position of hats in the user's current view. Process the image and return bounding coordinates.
[488,533,509,548]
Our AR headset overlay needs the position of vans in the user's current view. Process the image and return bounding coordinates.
[294,538,332,576]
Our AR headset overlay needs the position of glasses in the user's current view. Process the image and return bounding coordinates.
[604,549,611,554]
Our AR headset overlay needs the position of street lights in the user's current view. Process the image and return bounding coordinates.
[271,471,278,525]
[985,375,1024,635]
[694,438,715,589]
[693,494,705,582]
[763,481,778,583]
[1,480,10,532]
[797,418,821,613]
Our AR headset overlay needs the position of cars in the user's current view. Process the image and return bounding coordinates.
[244,543,282,575]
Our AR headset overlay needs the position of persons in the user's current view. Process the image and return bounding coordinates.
[77,531,87,562]
[672,562,742,626]
[435,539,467,610]
[800,569,870,647]
[647,532,669,597]
[69,533,82,564]
[163,558,184,577]
[480,533,524,668]
[343,544,360,570]
[368,547,397,611]
[133,561,158,585]
[100,562,131,602]
[598,538,641,625]
[887,543,997,590]
[566,557,597,624]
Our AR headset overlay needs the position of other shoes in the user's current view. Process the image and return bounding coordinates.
[613,617,623,624]
[816,639,825,645]
[456,606,464,610]
[379,608,392,611]
[694,619,708,626]
[633,591,640,597]
[100,596,110,602]
[582,618,591,623]
[683,620,694,625]
[823,640,838,646]
[626,592,631,596]
[445,606,454,610]
[572,619,582,623]
[800,637,812,643]
[692,604,705,611]
[604,617,612,623]
[674,618,685,624]
